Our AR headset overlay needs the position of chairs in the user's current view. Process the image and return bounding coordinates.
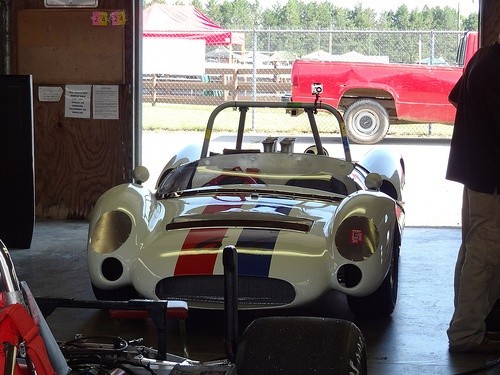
[203,166,265,188]
[283,174,349,199]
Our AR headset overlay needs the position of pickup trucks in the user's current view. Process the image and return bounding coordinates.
[287,31,479,145]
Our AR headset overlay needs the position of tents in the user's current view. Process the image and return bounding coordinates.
[142,1,234,64]
[208,42,445,92]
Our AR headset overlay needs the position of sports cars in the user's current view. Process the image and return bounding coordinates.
[82,101,403,325]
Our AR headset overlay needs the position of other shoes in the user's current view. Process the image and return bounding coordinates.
[484,331,499,339]
[477,337,500,352]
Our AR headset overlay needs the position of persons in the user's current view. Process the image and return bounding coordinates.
[443,33,500,354]
